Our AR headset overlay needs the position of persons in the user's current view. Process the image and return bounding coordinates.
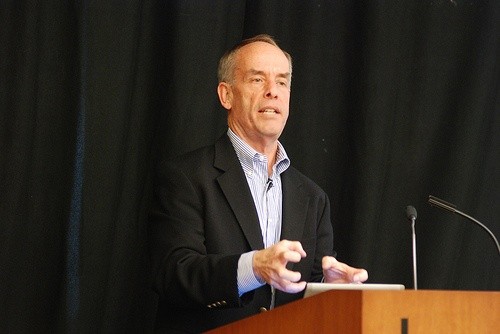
[145,31,369,334]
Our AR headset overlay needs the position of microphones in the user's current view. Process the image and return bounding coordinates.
[405,204,418,290]
[427,195,500,254]
[266,177,274,191]
